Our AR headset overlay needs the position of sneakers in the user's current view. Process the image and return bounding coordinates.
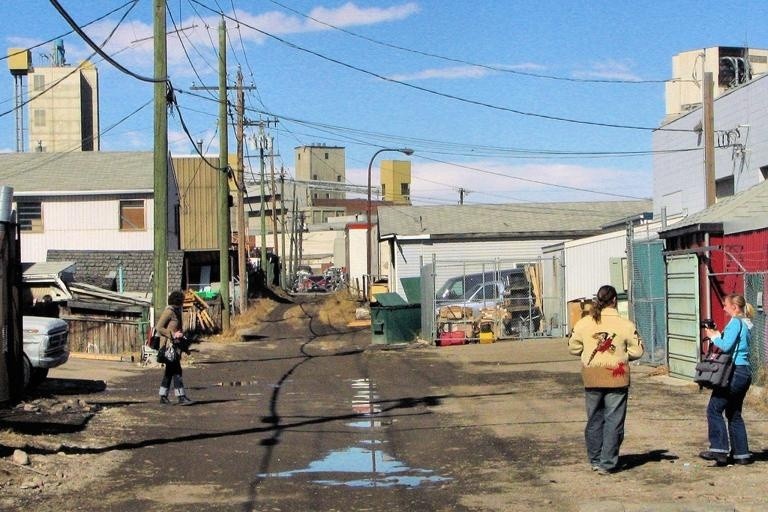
[178,398,194,405]
[159,399,171,404]
[698,451,728,464]
[727,454,754,465]
[590,463,624,476]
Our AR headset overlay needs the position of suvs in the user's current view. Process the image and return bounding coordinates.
[1,311,71,387]
[436,268,525,305]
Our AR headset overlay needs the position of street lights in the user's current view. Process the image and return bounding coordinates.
[367,146,413,303]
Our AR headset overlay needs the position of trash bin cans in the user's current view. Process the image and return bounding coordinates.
[370,277,421,345]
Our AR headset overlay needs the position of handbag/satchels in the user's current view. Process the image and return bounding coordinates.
[149,329,177,363]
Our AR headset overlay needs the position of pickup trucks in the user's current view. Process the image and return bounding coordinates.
[432,280,534,328]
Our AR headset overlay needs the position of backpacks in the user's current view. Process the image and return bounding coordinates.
[694,351,734,393]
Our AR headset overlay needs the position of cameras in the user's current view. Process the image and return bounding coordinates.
[700,318,716,330]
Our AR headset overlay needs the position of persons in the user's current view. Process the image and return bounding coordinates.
[699,293,755,465]
[34,294,60,319]
[568,285,644,475]
[156,291,195,407]
